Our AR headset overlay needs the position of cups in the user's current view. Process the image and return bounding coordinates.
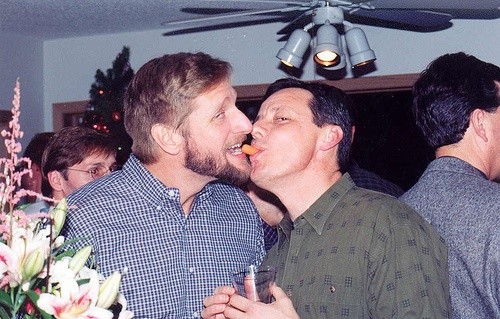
[229,266,276,304]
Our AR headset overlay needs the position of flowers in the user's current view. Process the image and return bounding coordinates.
[0,77,134,319]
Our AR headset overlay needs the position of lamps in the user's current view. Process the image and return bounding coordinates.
[313,20,342,68]
[342,20,376,69]
[276,23,314,69]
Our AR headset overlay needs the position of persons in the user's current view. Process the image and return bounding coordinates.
[397,51,500,319]
[200,78,452,319]
[243,118,405,251]
[0,109,119,225]
[38,52,267,319]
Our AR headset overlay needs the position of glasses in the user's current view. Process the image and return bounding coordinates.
[66,165,121,180]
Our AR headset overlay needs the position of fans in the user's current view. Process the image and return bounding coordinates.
[157,0,452,32]
